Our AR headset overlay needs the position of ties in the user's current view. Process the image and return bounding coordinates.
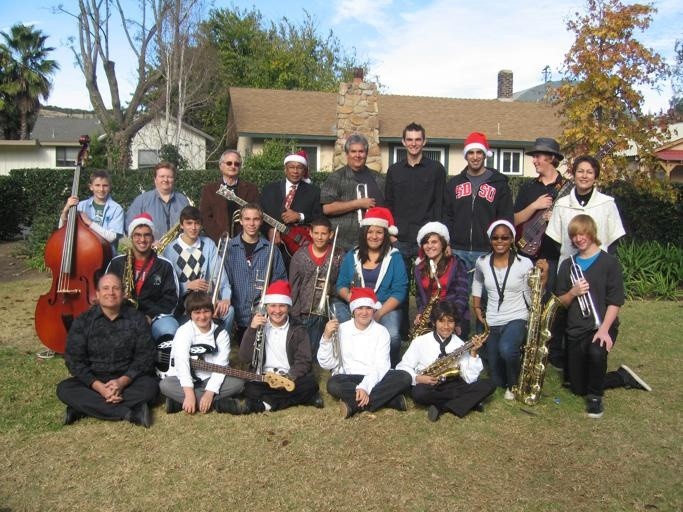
[283,185,297,211]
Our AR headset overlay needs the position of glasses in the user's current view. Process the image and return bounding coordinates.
[489,235,512,242]
[222,160,241,169]
[131,232,154,240]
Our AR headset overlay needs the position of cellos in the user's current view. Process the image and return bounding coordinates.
[34,136,115,354]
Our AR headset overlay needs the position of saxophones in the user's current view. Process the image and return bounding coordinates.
[150,192,196,258]
[122,239,137,310]
[416,304,490,383]
[407,258,441,339]
[511,259,568,406]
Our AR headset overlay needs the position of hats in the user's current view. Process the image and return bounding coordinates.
[524,137,567,161]
[263,279,294,308]
[349,287,383,316]
[417,219,450,246]
[486,219,517,242]
[360,206,399,237]
[463,133,495,159]
[129,214,155,238]
[284,150,312,185]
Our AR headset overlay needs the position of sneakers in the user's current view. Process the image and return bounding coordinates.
[616,363,652,393]
[36,348,63,360]
[583,397,605,419]
[503,389,515,404]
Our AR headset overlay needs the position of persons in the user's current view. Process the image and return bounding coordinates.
[410,220,470,342]
[237,278,325,415]
[314,286,413,421]
[162,204,236,343]
[392,299,497,423]
[468,217,551,403]
[55,168,126,259]
[255,150,322,278]
[554,214,653,420]
[103,212,182,341]
[330,206,410,369]
[53,270,160,430]
[157,290,246,416]
[198,148,261,247]
[121,160,191,251]
[536,154,627,371]
[444,131,515,336]
[285,215,347,364]
[220,201,290,347]
[382,121,449,342]
[319,134,385,254]
[512,137,575,307]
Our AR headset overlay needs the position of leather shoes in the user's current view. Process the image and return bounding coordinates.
[427,404,441,423]
[220,398,242,415]
[165,397,182,414]
[244,399,265,413]
[312,392,324,409]
[132,402,151,428]
[64,405,81,425]
[338,398,353,421]
[390,394,409,412]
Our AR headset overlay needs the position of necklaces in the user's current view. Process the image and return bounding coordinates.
[577,196,589,207]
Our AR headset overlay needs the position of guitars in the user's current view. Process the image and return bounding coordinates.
[514,137,618,257]
[151,334,295,388]
[215,182,343,259]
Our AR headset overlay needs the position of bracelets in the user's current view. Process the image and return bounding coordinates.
[473,306,482,309]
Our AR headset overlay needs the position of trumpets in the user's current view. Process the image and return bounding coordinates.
[568,255,602,334]
[250,308,268,383]
[324,295,345,376]
[355,183,368,224]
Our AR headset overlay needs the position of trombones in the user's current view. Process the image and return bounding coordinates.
[205,231,228,320]
[307,224,339,361]
[241,223,278,373]
